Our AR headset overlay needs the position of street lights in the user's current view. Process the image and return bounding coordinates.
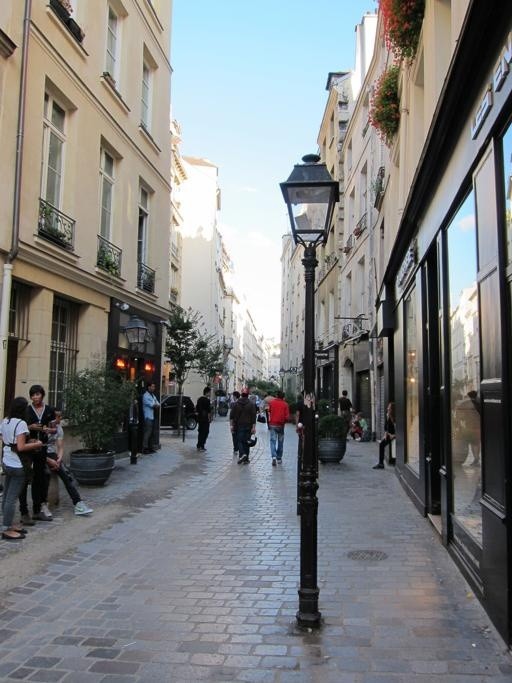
[279,366,286,393]
[223,371,230,394]
[278,151,344,632]
[268,373,277,385]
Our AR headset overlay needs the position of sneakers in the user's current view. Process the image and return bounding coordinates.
[373,463,384,469]
[75,501,93,514]
[238,454,249,465]
[272,457,277,465]
[278,460,282,464]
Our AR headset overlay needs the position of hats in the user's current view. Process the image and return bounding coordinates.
[240,388,249,394]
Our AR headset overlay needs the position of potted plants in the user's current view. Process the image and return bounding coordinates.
[317,412,352,465]
[59,366,144,488]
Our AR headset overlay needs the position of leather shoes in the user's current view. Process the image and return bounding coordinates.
[2,503,52,539]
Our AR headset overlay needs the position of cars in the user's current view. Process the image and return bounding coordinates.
[161,392,197,430]
[248,394,261,409]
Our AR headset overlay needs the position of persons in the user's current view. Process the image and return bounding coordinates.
[456,394,480,467]
[41,406,94,519]
[339,390,355,442]
[141,381,162,455]
[350,411,366,440]
[18,384,58,526]
[229,391,290,466]
[196,387,212,452]
[295,390,304,433]
[371,402,395,469]
[0,397,43,540]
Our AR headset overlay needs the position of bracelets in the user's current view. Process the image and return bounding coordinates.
[33,439,36,442]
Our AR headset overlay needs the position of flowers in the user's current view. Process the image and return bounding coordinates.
[365,66,409,146]
[376,0,426,65]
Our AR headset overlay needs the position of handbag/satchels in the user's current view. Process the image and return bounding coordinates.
[257,415,266,422]
[247,433,257,447]
